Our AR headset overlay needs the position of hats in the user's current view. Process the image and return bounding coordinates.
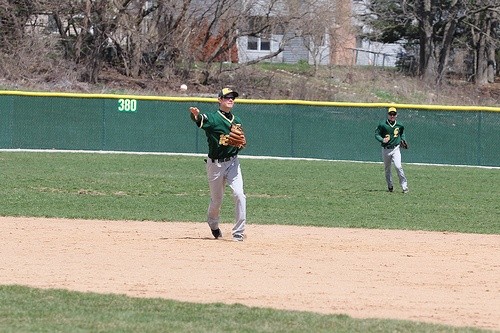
[388,106,396,112]
[218,87,239,97]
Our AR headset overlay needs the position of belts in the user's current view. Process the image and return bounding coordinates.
[384,142,399,149]
[211,154,236,162]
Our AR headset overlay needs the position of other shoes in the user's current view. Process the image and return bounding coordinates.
[402,186,410,193]
[232,233,243,241]
[388,184,393,191]
[211,228,222,238]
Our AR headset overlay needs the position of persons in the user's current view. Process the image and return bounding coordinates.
[189,87,246,242]
[375,108,408,193]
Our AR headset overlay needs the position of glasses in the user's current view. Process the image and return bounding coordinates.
[220,96,235,99]
[388,112,397,115]
